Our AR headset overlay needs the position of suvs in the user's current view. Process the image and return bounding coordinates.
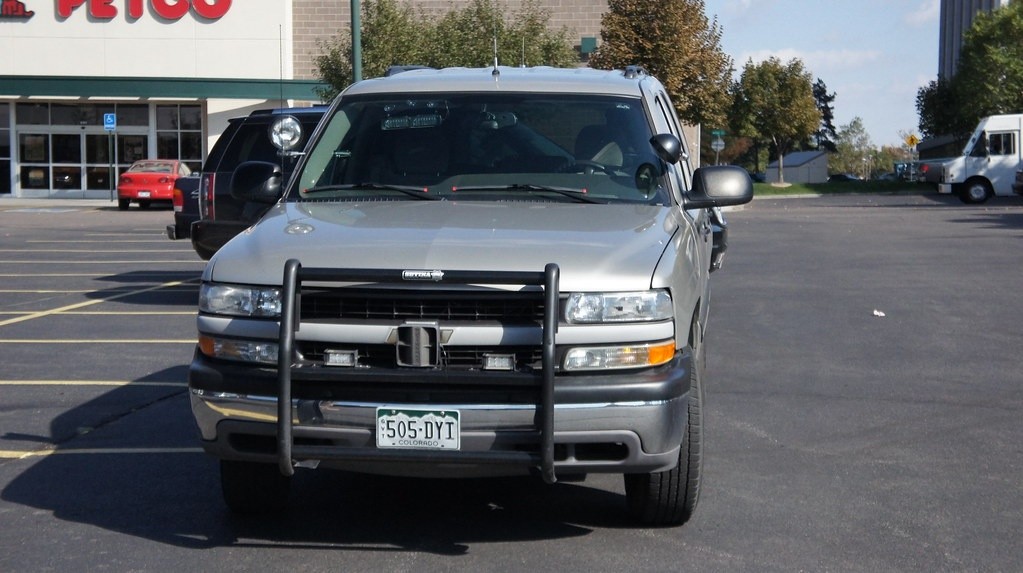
[166,104,332,261]
[191,63,756,527]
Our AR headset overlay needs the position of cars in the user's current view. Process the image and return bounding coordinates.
[117,160,190,211]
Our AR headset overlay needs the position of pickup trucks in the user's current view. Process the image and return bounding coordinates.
[916,156,954,195]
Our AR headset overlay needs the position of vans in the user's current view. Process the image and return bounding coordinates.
[943,114,1023,205]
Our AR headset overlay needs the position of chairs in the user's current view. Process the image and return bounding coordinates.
[381,127,456,188]
[574,123,610,160]
